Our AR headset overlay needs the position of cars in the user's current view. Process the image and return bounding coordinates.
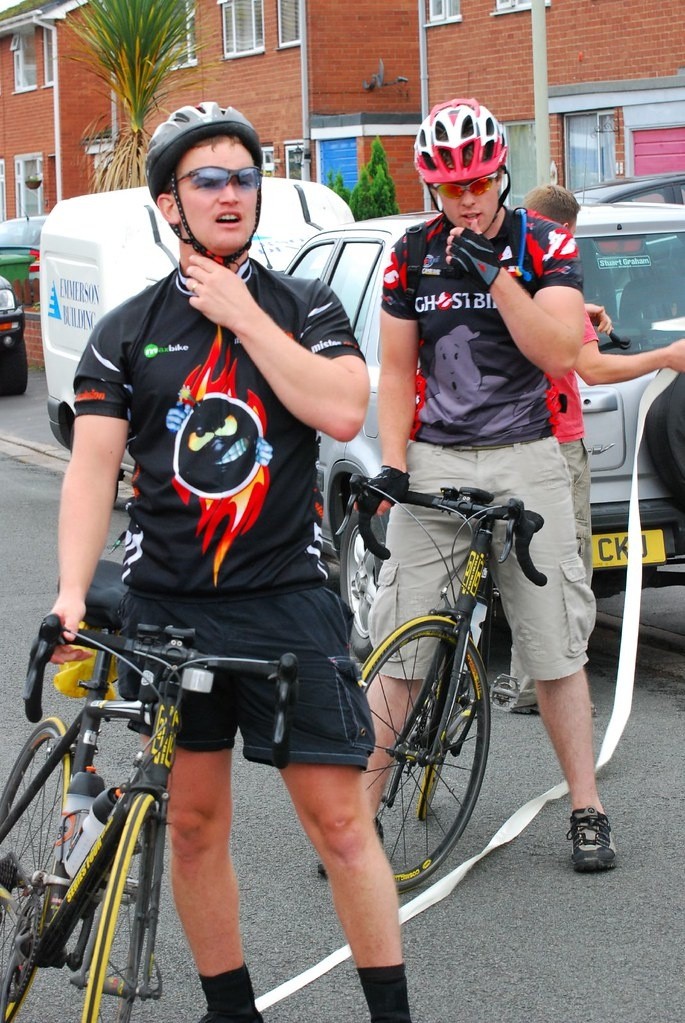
[0,216,53,396]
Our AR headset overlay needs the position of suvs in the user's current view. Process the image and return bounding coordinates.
[281,175,684,624]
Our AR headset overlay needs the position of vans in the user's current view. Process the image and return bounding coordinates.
[38,175,351,502]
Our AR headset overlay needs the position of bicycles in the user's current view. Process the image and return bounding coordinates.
[335,474,549,894]
[1,558,299,1022]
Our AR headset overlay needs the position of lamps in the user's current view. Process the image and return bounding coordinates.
[291,142,304,169]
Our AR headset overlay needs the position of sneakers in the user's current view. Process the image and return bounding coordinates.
[318,817,384,877]
[566,805,615,870]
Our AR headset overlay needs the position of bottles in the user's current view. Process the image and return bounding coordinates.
[64,787,122,879]
[464,589,488,671]
[55,766,106,863]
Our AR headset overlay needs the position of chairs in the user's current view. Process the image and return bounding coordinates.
[613,276,674,325]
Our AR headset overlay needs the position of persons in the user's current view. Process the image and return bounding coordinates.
[309,99,620,876]
[40,104,415,1023]
[508,183,685,717]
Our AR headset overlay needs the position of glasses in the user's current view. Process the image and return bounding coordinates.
[430,170,499,199]
[165,166,264,192]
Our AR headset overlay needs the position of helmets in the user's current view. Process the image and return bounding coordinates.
[414,98,509,183]
[145,102,264,203]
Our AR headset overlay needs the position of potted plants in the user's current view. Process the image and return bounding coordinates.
[24,174,42,190]
